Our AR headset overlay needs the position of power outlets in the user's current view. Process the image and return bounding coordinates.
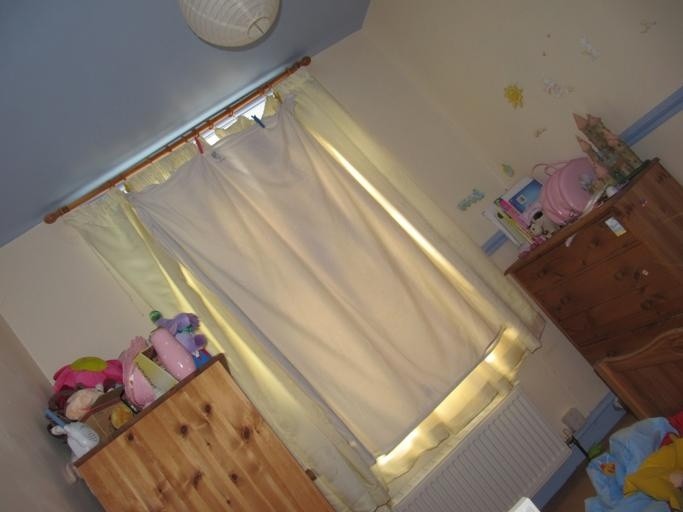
[563,407,587,433]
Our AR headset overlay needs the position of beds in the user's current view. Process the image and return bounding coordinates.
[593,327,683,512]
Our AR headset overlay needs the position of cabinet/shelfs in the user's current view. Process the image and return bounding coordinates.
[503,156,683,421]
[72,352,337,512]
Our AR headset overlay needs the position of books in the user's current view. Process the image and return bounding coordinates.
[482,176,560,251]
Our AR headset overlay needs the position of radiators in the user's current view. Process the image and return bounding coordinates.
[392,383,573,511]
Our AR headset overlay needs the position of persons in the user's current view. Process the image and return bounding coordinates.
[526,211,553,239]
[572,111,650,188]
